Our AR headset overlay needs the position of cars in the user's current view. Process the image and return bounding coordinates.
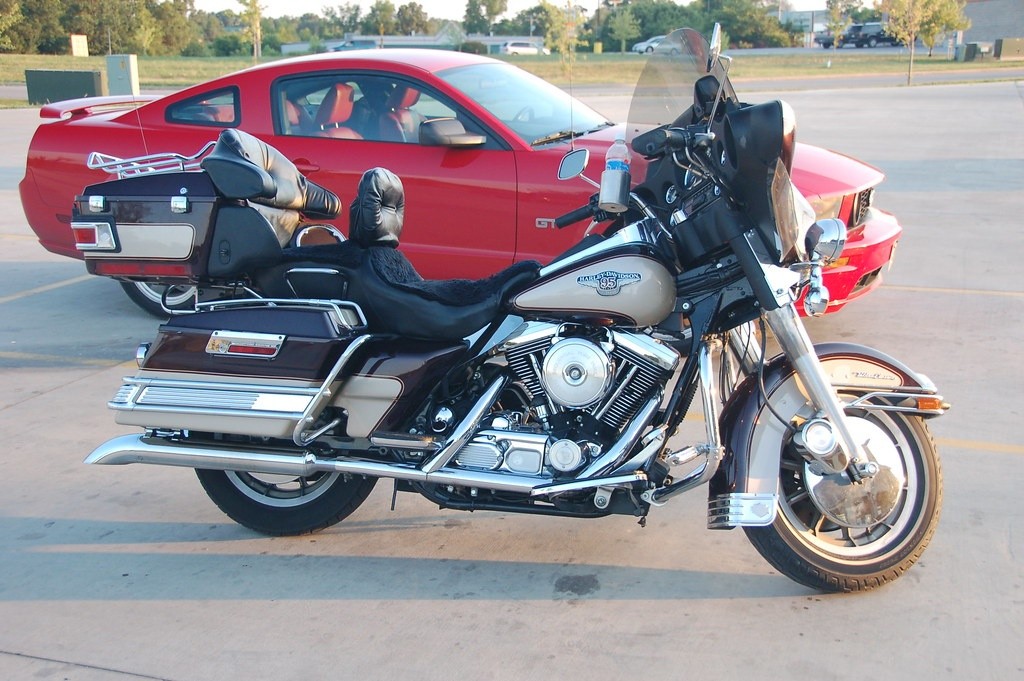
[815,21,906,49]
[500,40,552,57]
[632,35,685,56]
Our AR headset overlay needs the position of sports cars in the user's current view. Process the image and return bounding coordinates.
[19,48,902,318]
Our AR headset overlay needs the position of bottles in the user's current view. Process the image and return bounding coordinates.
[605,136,631,172]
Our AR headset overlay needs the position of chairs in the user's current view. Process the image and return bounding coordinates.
[313,82,364,139]
[379,84,429,143]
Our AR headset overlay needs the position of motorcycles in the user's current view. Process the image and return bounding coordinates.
[69,19,951,596]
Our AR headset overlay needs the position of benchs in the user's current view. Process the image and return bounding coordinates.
[193,100,302,127]
